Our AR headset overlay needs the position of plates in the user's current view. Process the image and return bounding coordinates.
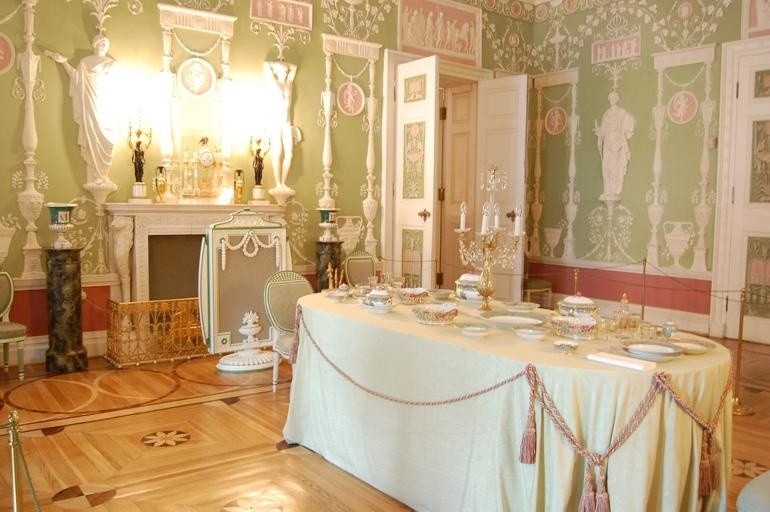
[665,335,717,355]
[462,327,488,336]
[477,308,551,328]
[503,300,541,312]
[621,337,682,363]
[514,327,550,340]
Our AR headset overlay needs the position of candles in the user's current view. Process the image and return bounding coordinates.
[480,201,490,234]
[494,203,501,228]
[514,204,524,236]
[460,201,468,231]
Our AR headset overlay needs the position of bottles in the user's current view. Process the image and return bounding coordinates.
[155,165,168,204]
[234,168,246,205]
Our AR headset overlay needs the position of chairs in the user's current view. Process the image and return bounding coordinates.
[736,470,770,512]
[263,271,314,398]
[523,237,553,309]
[1,272,27,382]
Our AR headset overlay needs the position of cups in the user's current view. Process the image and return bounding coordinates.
[598,291,678,350]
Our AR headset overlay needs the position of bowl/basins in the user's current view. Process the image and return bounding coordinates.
[553,342,579,354]
[321,270,484,325]
[316,207,341,224]
[555,291,601,319]
[46,203,78,225]
[548,313,598,340]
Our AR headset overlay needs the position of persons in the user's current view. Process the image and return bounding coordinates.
[51,35,121,186]
[595,91,635,198]
[261,59,298,188]
[132,141,146,182]
[252,148,265,185]
[402,6,476,56]
